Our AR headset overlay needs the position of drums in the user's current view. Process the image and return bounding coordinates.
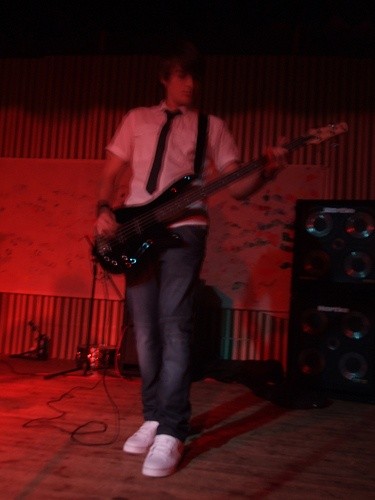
[75,344,116,369]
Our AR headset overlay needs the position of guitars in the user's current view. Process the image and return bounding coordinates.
[91,122,350,275]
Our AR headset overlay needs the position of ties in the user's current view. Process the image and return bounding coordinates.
[145,109,183,195]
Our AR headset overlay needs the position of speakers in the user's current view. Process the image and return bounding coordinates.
[286,286,375,406]
[291,199,375,285]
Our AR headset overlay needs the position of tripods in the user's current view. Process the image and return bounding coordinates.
[43,226,121,378]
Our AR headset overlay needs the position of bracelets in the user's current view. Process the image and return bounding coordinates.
[260,170,274,182]
[95,199,113,217]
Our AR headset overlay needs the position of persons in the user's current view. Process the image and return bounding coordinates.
[91,43,291,478]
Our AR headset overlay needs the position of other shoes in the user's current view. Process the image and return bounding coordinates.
[124,420,184,477]
